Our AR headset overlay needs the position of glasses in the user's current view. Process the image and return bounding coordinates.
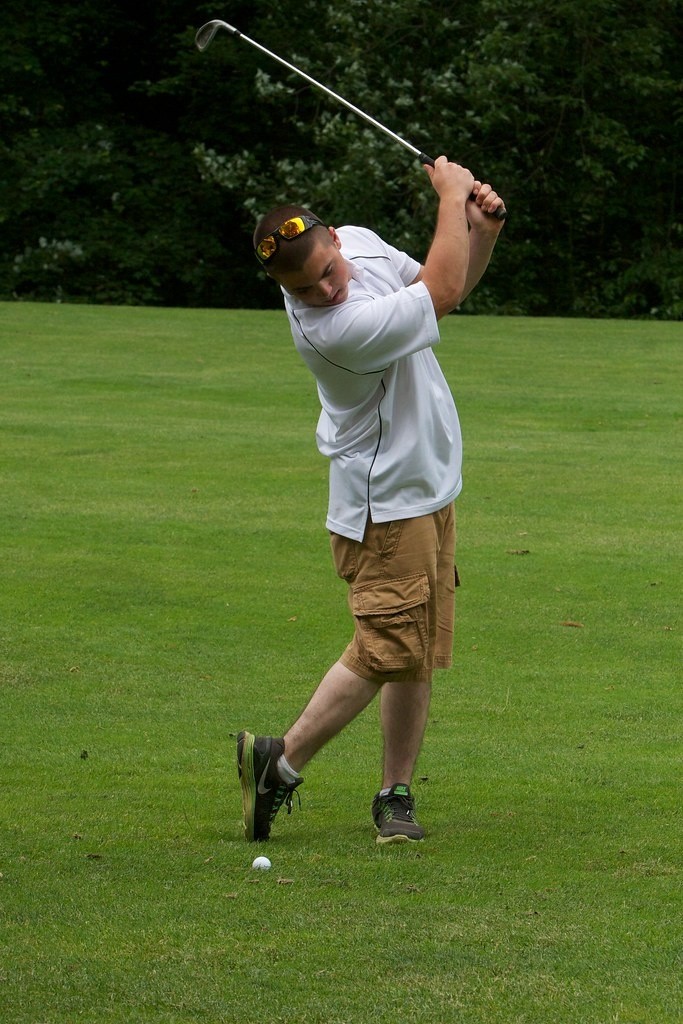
[252,215,329,265]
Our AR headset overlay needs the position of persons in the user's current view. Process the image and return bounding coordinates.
[236,156,506,845]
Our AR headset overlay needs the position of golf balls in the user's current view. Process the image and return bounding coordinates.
[251,856,271,870]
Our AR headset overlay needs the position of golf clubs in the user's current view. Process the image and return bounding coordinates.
[195,19,508,221]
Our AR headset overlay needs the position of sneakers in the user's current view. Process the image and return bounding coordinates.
[371,783,425,844]
[237,730,304,843]
